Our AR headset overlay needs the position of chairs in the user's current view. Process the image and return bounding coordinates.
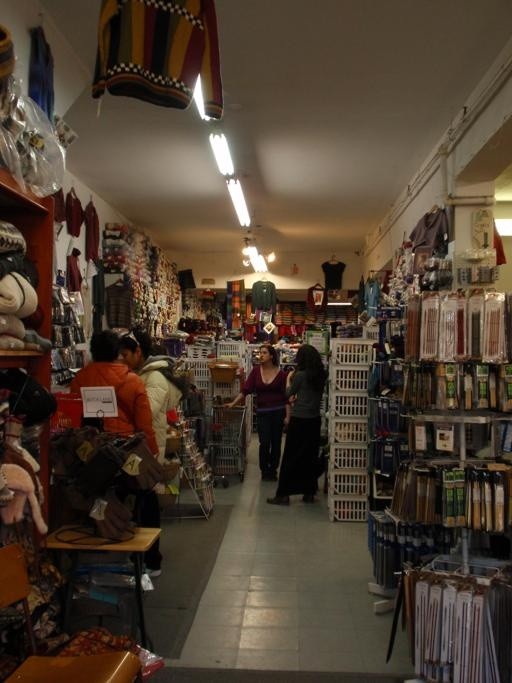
[1,537,144,682]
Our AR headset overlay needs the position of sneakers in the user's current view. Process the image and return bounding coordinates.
[266,495,289,506]
[301,495,314,504]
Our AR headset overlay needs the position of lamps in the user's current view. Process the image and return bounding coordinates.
[189,71,275,277]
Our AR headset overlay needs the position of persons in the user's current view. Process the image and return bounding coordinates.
[226,343,291,481]
[121,328,182,578]
[69,329,159,460]
[268,344,328,505]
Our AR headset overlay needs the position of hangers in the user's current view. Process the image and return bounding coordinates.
[311,279,324,289]
[359,268,380,285]
[327,252,340,263]
[112,272,127,287]
[85,190,98,208]
[69,176,80,201]
[24,10,54,54]
[426,202,440,217]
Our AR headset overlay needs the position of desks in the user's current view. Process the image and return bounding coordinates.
[37,521,164,663]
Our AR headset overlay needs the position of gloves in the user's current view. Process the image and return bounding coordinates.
[50,426,164,540]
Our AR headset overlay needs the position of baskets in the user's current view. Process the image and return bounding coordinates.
[181,342,252,476]
[321,338,369,523]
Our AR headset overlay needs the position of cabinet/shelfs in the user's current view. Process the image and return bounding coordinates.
[0,164,52,657]
[167,306,398,522]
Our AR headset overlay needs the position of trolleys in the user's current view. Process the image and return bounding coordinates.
[205,401,247,487]
